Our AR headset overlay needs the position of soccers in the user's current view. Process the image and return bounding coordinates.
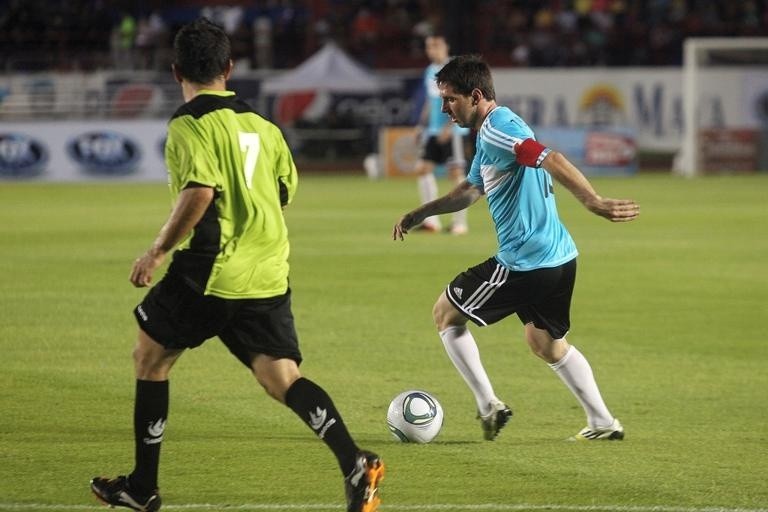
[387,392,445,445]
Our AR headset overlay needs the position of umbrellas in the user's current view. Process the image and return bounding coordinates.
[260,42,399,165]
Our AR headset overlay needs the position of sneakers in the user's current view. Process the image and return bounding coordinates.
[564,418,624,444]
[339,449,386,512]
[408,223,470,235]
[475,400,514,441]
[89,476,162,512]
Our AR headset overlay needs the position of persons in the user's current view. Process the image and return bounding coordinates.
[393,56,639,443]
[413,30,472,234]
[90,16,384,512]
